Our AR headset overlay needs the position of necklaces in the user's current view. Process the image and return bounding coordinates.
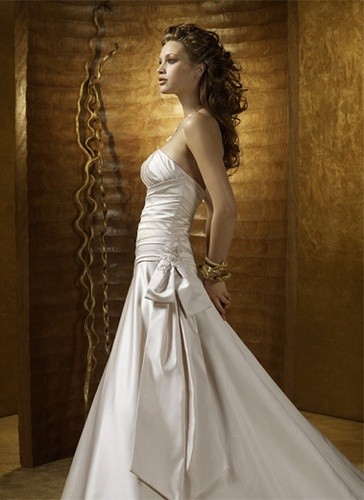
[166,108,201,142]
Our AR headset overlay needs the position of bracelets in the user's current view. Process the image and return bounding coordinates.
[201,257,231,282]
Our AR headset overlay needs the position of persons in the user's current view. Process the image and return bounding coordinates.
[60,22,364,500]
